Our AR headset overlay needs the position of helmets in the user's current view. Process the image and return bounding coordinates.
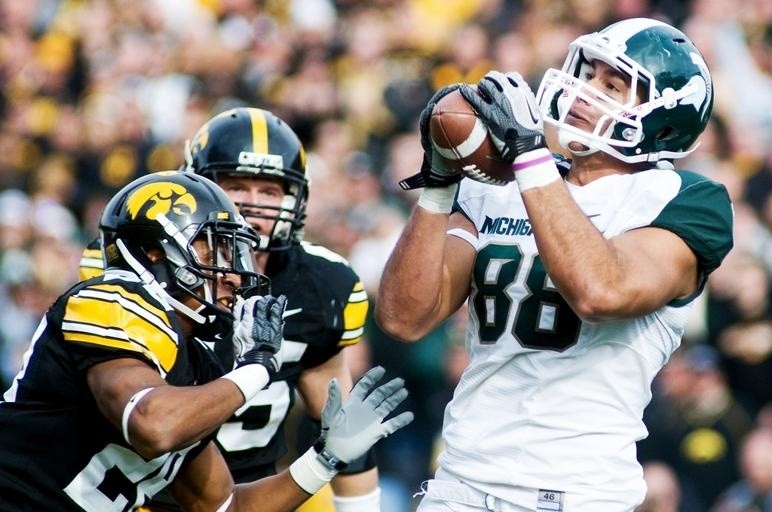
[180,104,310,253]
[535,16,714,165]
[97,165,260,341]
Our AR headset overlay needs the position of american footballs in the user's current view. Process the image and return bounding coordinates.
[429,85,515,186]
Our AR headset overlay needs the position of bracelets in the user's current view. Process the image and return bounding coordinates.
[508,153,554,171]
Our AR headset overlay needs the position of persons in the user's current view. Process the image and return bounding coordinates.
[0,169,416,512]
[79,105,382,512]
[370,16,735,511]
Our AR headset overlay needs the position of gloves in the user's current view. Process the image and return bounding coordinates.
[287,363,414,497]
[416,78,465,216]
[458,69,561,193]
[221,294,290,407]
